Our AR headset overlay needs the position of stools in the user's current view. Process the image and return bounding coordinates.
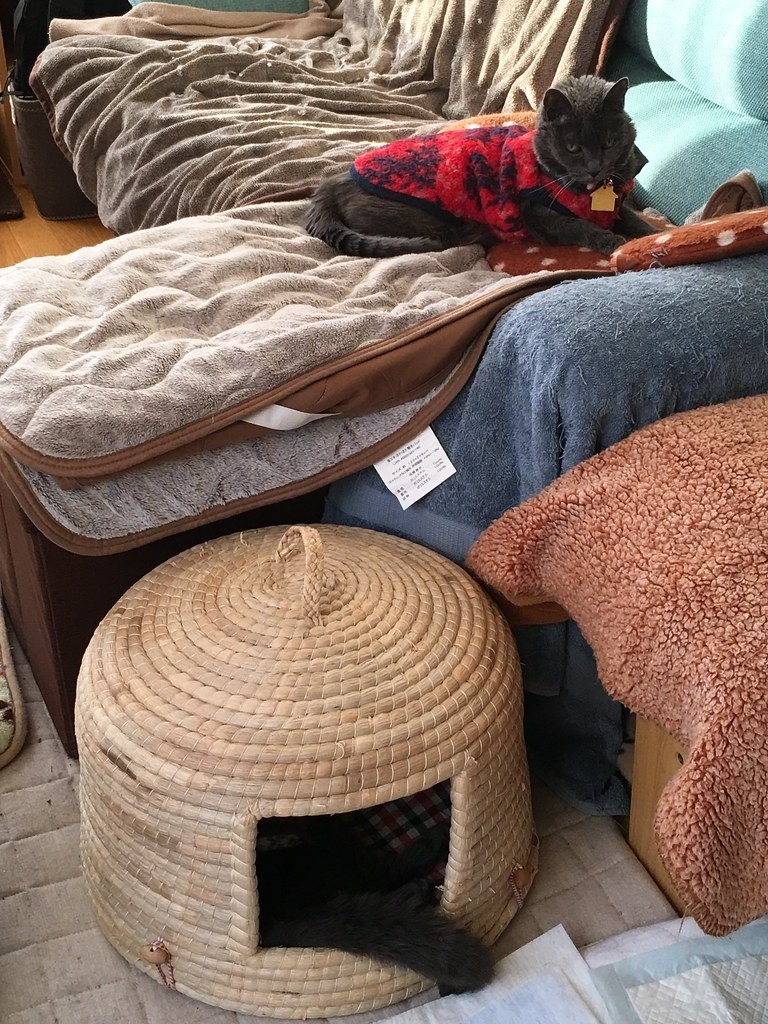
[463,391,768,939]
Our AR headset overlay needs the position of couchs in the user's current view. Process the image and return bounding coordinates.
[0,0,768,818]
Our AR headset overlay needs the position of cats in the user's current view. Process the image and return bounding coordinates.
[304,70,667,258]
[261,888,494,992]
